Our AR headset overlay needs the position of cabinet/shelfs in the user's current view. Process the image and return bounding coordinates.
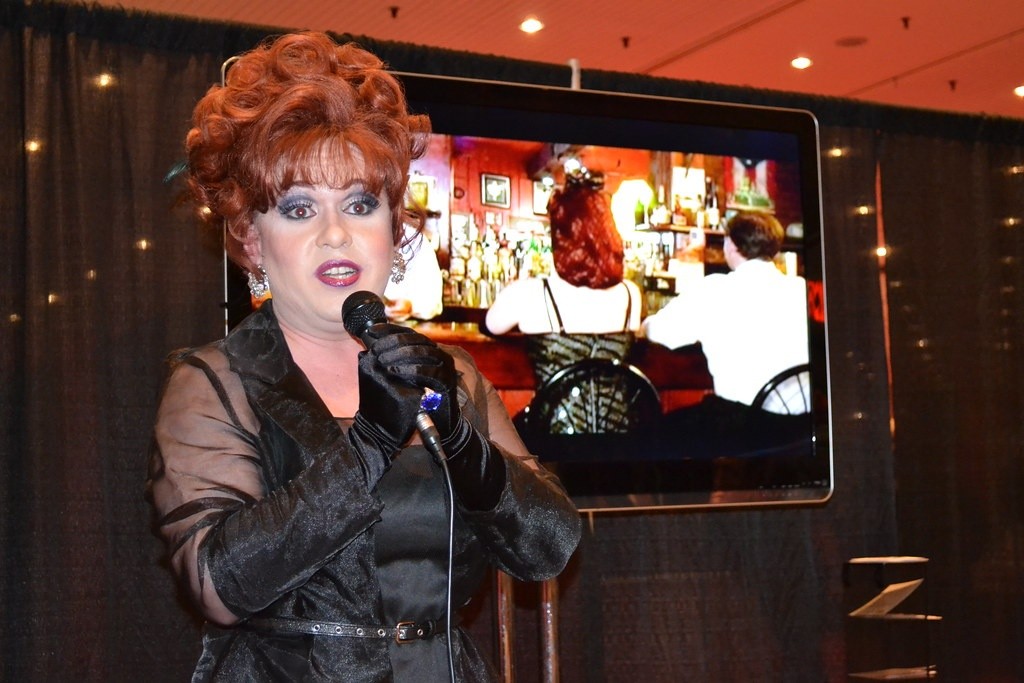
[843,555,932,683]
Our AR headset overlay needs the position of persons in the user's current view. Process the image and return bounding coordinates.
[479,182,642,434]
[146,33,582,683]
[635,210,812,458]
[384,225,443,322]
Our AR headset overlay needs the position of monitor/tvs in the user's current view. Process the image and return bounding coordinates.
[219,55,834,516]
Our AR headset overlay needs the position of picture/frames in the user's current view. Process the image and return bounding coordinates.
[482,173,510,210]
[533,180,548,215]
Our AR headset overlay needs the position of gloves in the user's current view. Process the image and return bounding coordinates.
[349,351,425,495]
[368,323,507,511]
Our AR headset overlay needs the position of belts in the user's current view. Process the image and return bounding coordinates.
[247,611,466,647]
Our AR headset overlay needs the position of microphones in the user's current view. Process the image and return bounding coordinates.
[340,290,448,466]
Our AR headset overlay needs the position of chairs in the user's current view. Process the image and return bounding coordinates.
[523,358,664,439]
[741,362,814,470]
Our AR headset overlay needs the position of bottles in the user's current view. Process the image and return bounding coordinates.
[447,176,780,310]
